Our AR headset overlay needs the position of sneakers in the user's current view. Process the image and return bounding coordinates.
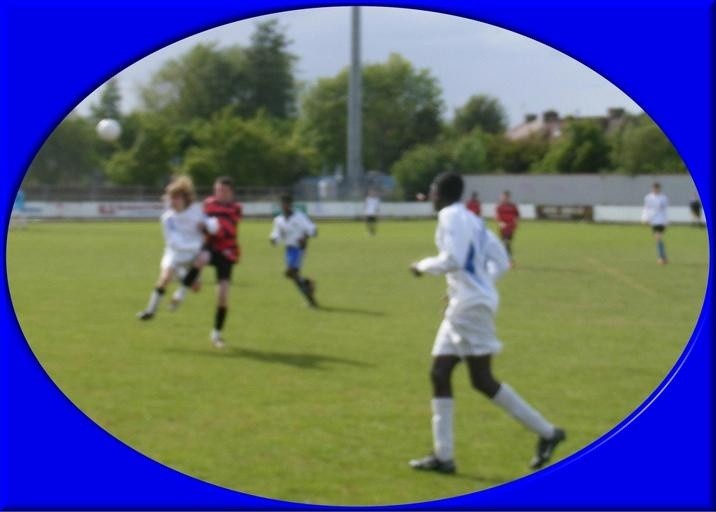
[408,452,458,474]
[529,426,567,469]
[302,279,318,308]
[135,277,226,349]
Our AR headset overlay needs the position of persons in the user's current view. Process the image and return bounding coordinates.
[362,185,382,242]
[170,179,240,350]
[408,171,566,471]
[496,190,521,268]
[465,190,481,213]
[688,196,702,231]
[268,194,319,309]
[134,175,216,321]
[639,181,673,267]
